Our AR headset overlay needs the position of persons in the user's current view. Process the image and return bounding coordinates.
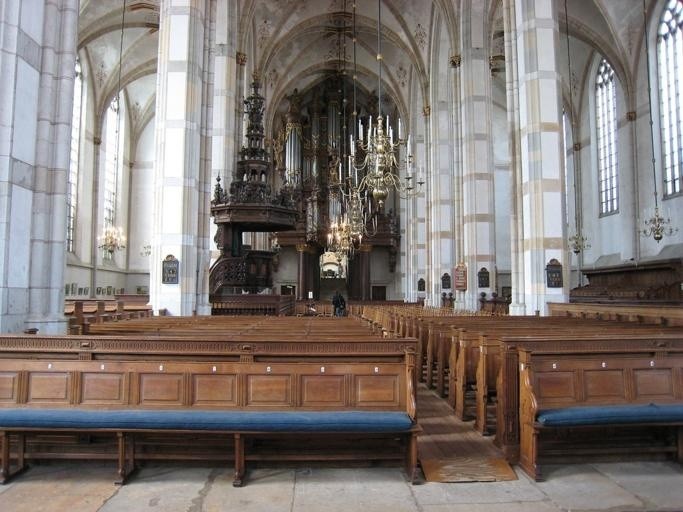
[335,292,345,317]
[331,290,339,317]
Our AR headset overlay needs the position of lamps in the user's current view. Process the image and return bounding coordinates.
[318,0,424,280]
[236,52,247,66]
[96,0,128,252]
[564,0,591,255]
[448,54,461,68]
[635,0,681,243]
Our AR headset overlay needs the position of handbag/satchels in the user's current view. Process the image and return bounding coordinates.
[338,295,344,309]
